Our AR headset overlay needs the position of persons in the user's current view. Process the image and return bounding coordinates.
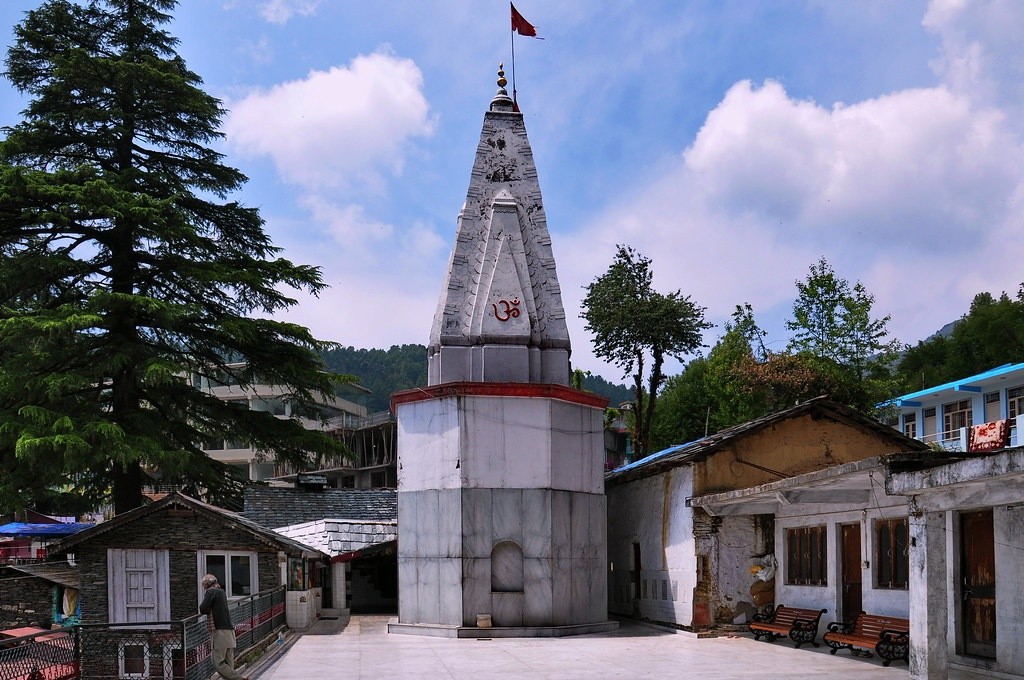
[199,574,250,680]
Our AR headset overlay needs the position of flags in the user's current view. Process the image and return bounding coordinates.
[511,3,536,37]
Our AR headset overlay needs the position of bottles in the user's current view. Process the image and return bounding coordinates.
[277,635,283,645]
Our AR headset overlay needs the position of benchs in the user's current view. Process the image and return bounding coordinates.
[749,604,909,667]
